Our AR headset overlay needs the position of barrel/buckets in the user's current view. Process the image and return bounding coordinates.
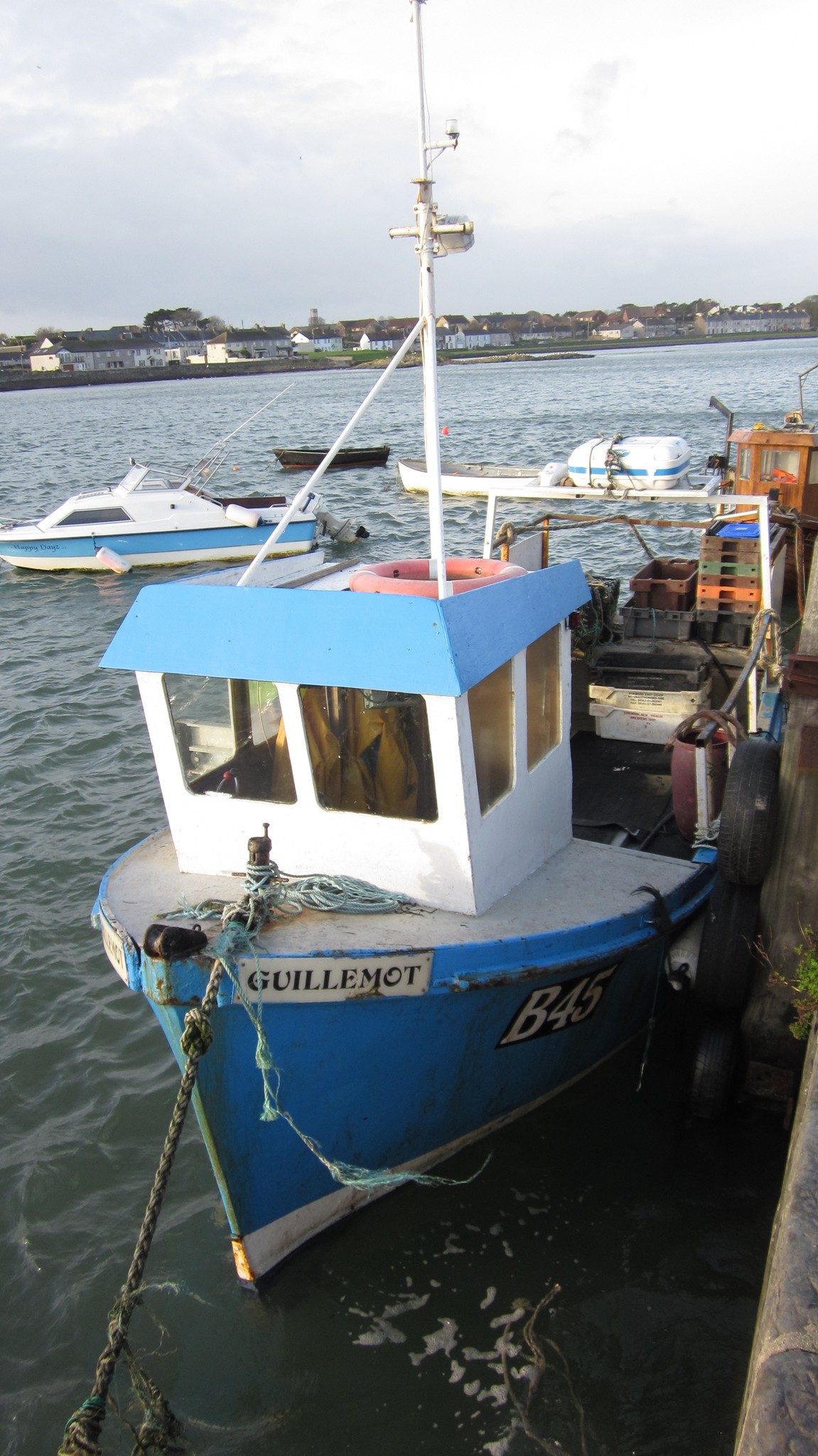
[670,730,727,842]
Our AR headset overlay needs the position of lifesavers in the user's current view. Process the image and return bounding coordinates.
[693,879,760,1016]
[350,555,528,600]
[686,1016,738,1121]
[716,736,781,888]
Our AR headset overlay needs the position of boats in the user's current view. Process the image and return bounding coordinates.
[96,0,727,1290]
[0,454,319,576]
[399,434,723,497]
[272,444,390,471]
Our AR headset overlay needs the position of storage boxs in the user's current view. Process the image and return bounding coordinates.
[588,520,762,744]
